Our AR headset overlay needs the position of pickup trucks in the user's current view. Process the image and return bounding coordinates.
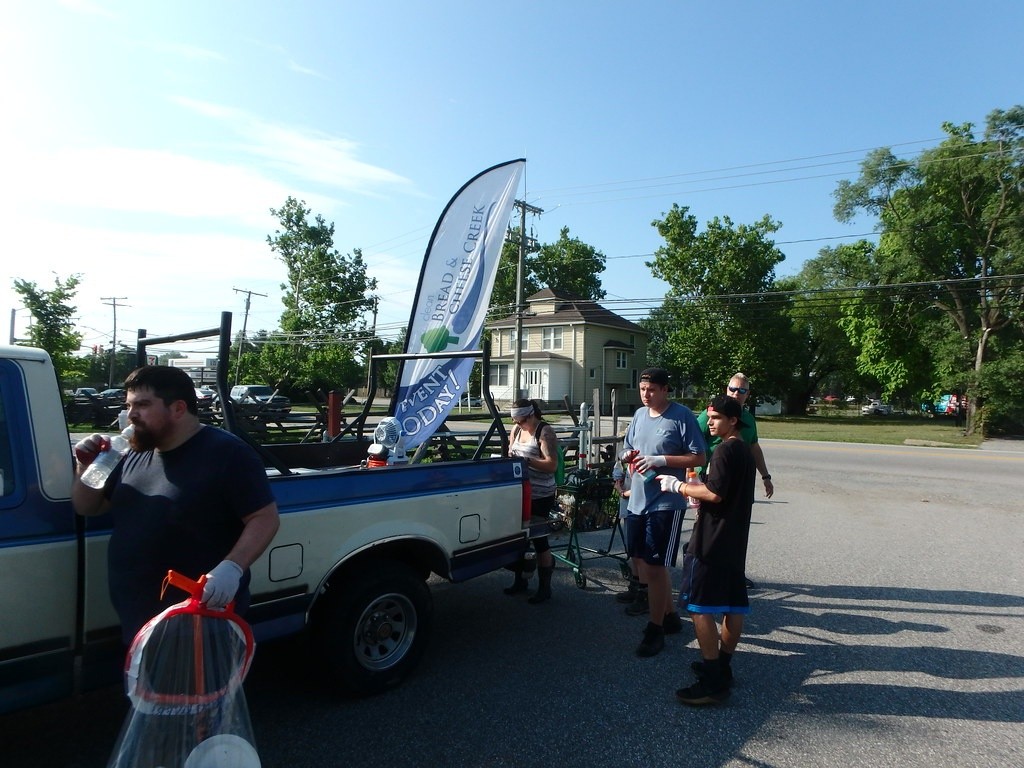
[1,343,538,722]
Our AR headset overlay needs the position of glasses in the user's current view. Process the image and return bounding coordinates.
[727,386,748,395]
[512,409,534,424]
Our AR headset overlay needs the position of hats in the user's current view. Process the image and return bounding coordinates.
[708,394,752,431]
[640,367,675,392]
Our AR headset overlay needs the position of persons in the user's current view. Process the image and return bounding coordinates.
[612,459,650,615]
[682,372,773,589]
[618,368,707,658]
[73,365,282,768]
[503,398,558,604]
[655,395,756,705]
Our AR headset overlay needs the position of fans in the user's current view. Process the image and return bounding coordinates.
[372,416,406,458]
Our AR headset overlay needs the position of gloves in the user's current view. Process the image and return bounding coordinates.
[200,560,243,611]
[618,449,641,463]
[74,434,111,474]
[655,474,683,492]
[633,455,668,474]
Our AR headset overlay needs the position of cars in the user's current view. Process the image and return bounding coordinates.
[74,386,220,407]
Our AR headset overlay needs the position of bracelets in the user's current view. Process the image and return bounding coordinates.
[623,492,628,499]
[683,484,688,495]
[762,475,771,480]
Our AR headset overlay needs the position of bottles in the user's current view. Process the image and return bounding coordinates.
[80,424,135,489]
[688,472,701,507]
[632,455,656,482]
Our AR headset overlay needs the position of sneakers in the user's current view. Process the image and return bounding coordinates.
[676,677,725,705]
[616,577,639,603]
[691,661,733,680]
[664,615,682,633]
[635,621,664,657]
[626,588,649,615]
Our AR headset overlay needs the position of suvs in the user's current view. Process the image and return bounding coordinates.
[230,382,291,418]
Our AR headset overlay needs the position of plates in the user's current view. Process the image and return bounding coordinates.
[184,734,262,768]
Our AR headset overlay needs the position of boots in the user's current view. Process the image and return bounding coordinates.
[537,556,555,597]
[502,570,528,595]
